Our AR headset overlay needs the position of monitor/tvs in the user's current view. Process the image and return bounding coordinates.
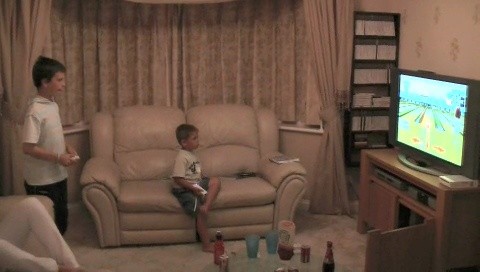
[388,67,480,180]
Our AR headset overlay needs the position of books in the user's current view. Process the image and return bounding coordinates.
[353,69,391,87]
[352,92,391,111]
[351,115,393,133]
[270,155,301,164]
[354,43,397,64]
[354,17,396,39]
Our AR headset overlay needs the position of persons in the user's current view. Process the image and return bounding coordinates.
[170,122,222,259]
[22,55,80,247]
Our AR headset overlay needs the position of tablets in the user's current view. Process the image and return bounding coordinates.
[269,154,299,163]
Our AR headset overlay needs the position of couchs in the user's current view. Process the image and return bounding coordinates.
[0,195,58,265]
[79,104,197,248]
[187,104,309,241]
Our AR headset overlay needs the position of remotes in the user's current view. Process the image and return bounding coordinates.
[193,183,207,196]
[237,172,257,177]
[73,156,80,159]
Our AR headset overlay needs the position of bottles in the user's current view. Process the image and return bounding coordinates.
[214,231,225,264]
[322,239,335,272]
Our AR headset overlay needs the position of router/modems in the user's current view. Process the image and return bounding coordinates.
[440,174,473,189]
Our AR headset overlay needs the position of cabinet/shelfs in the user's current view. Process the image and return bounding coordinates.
[357,149,477,271]
[346,11,401,167]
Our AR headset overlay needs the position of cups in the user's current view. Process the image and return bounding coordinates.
[265,231,278,254]
[277,220,296,262]
[245,234,260,258]
